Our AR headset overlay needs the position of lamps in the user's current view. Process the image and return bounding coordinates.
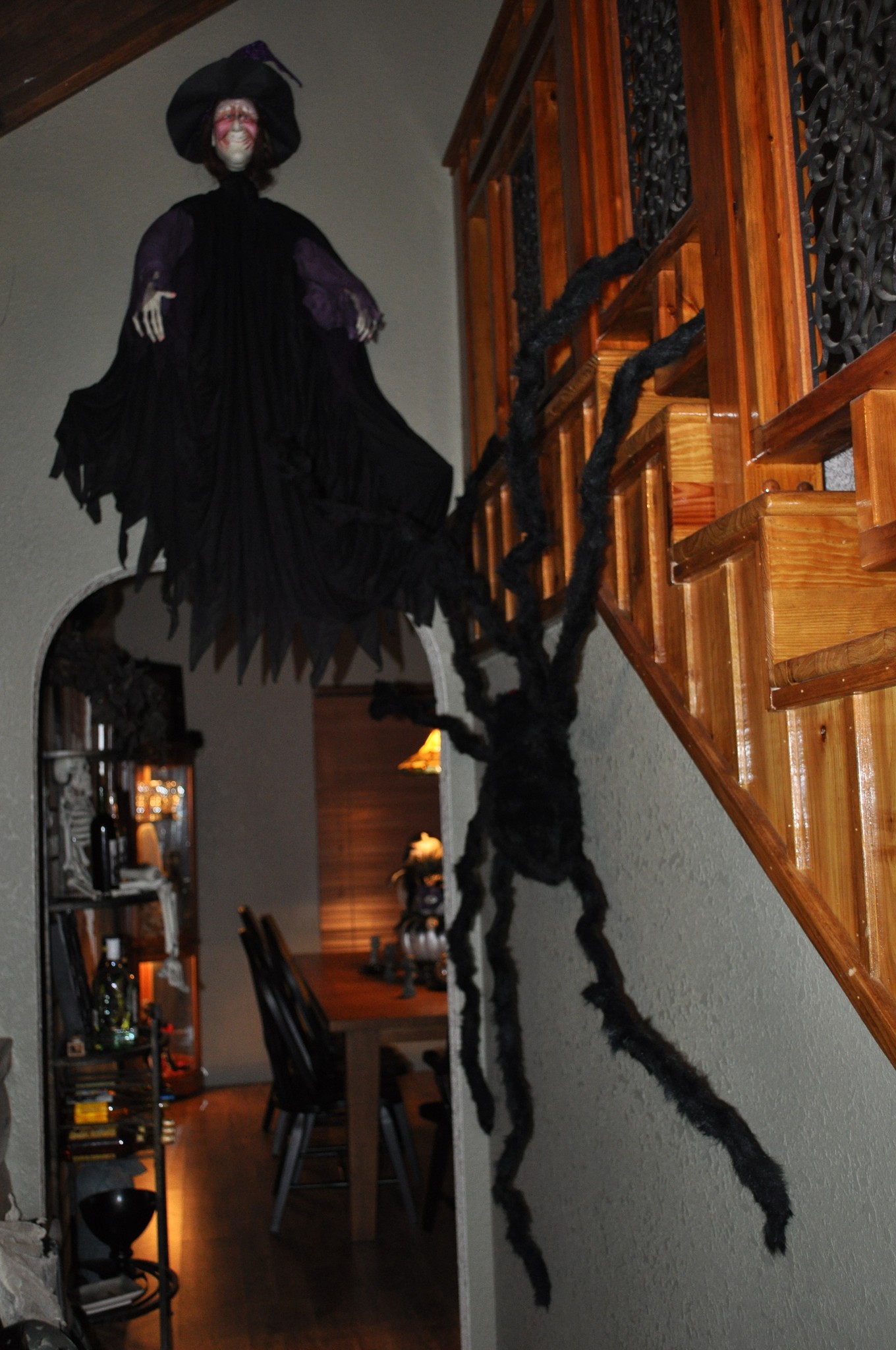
[397,728,442,774]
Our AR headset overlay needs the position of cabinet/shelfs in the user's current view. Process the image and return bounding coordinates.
[89,727,204,1109]
[38,746,179,1350]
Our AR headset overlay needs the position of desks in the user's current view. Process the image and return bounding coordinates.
[293,952,448,1244]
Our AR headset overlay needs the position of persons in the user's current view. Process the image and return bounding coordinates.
[48,39,455,690]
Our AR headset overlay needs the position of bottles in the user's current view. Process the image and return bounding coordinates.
[89,936,136,1053]
[90,786,121,892]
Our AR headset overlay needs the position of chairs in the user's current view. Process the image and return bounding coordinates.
[238,904,419,1239]
